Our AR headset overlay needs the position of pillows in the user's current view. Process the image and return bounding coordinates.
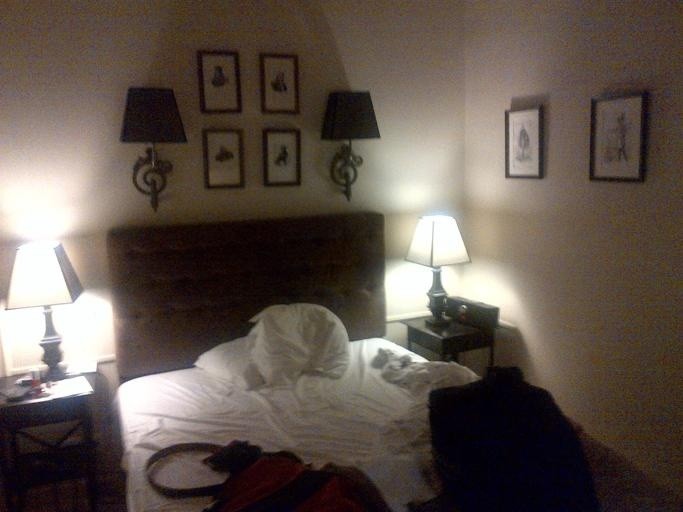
[192,335,255,392]
[247,301,349,386]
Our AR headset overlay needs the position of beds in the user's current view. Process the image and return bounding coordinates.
[93,210,583,511]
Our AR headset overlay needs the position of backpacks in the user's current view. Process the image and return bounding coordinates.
[204,448,389,511]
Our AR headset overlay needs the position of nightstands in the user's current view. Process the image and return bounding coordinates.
[0,361,95,511]
[401,315,522,367]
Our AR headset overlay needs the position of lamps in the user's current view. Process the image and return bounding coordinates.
[404,215,471,328]
[120,87,188,211]
[320,89,381,200]
[5,240,84,381]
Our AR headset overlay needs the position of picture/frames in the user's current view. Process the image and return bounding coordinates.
[197,49,242,114]
[263,127,302,187]
[504,103,546,179]
[201,128,245,189]
[589,90,647,183]
[260,53,300,115]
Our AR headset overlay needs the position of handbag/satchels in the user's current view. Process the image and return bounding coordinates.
[425,362,605,512]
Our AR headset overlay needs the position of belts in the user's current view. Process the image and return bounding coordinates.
[143,441,227,494]
